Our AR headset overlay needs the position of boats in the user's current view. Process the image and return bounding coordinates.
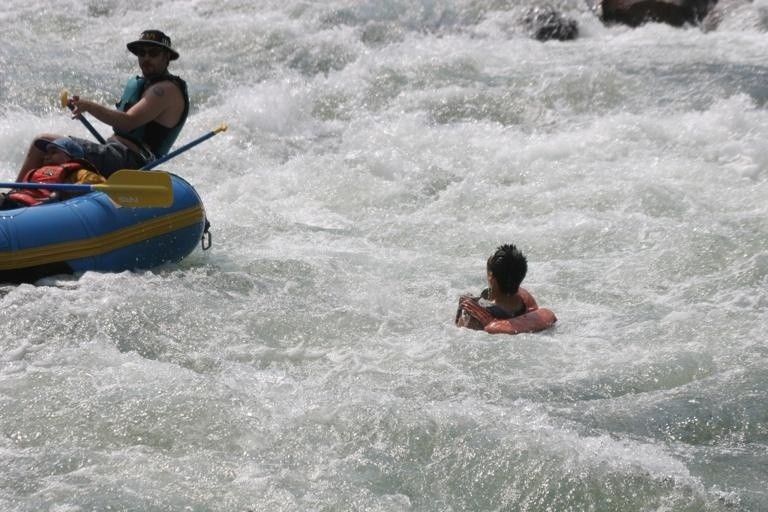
[0,168,215,284]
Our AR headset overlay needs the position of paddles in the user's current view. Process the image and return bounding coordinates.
[0,170,174,209]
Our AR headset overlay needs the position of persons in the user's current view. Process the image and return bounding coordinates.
[8,29,189,190]
[456,242,539,329]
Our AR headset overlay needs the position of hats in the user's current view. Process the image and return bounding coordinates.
[33,136,85,159]
[126,29,179,60]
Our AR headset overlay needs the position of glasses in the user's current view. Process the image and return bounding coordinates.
[136,46,166,57]
